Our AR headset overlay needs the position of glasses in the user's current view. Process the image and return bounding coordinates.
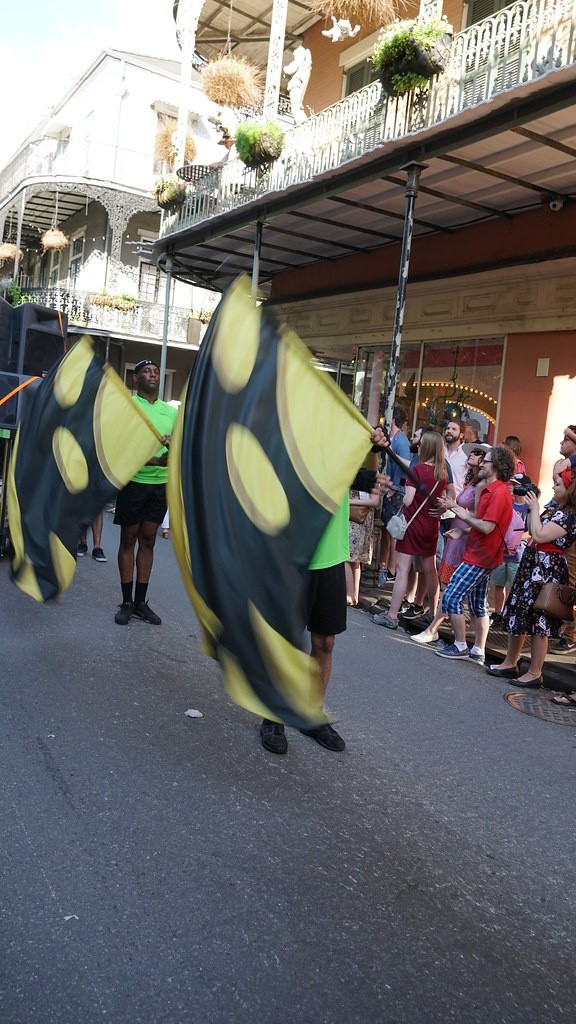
[471,450,481,456]
[478,457,492,464]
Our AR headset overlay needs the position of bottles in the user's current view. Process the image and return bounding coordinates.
[378,563,387,587]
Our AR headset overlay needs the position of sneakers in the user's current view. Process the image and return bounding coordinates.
[549,637,576,654]
[77,543,88,556]
[115,601,133,625]
[399,598,424,619]
[260,718,287,753]
[300,723,345,751]
[372,611,399,629]
[435,644,470,659]
[470,652,485,666]
[92,547,107,561]
[132,599,161,625]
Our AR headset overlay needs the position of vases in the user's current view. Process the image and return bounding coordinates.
[378,34,452,98]
[158,191,186,211]
[187,318,209,345]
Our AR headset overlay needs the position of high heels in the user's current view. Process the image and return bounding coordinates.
[162,528,169,539]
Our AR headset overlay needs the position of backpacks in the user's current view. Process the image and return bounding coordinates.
[504,508,524,557]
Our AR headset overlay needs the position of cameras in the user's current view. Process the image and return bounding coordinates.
[513,473,539,498]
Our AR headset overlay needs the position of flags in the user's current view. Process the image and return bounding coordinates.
[167,269,379,728]
[5,331,166,601]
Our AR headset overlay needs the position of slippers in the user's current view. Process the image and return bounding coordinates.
[551,694,576,705]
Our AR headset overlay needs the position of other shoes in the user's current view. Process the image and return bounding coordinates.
[410,632,439,643]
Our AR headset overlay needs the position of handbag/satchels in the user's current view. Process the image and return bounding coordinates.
[533,583,576,621]
[386,514,407,540]
[350,505,370,523]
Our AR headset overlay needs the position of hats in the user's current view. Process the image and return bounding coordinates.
[509,473,531,485]
[135,359,159,373]
[462,443,492,458]
[465,419,481,431]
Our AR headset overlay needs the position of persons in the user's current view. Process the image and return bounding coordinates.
[485,425,576,705]
[260,428,389,754]
[77,512,107,562]
[113,360,179,624]
[345,406,492,643]
[283,45,312,125]
[208,113,233,137]
[410,435,530,664]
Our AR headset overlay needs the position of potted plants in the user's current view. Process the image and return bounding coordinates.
[234,120,286,168]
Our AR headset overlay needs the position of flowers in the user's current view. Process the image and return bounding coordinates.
[190,307,215,324]
[366,15,453,99]
[150,176,186,207]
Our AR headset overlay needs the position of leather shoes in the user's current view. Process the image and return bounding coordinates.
[508,674,543,688]
[486,666,519,677]
